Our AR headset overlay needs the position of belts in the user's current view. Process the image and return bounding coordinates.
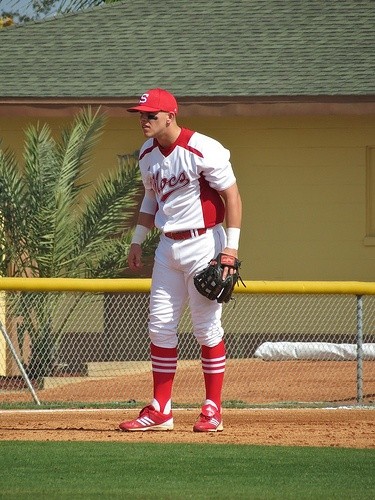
[165,228,206,240]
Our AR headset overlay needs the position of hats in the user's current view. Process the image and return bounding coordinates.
[126,88,177,114]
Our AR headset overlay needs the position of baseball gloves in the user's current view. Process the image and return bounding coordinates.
[192,253,246,303]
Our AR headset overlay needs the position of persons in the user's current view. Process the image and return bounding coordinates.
[119,89,243,433]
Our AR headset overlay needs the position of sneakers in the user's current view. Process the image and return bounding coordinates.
[192,404,223,431]
[119,405,173,431]
